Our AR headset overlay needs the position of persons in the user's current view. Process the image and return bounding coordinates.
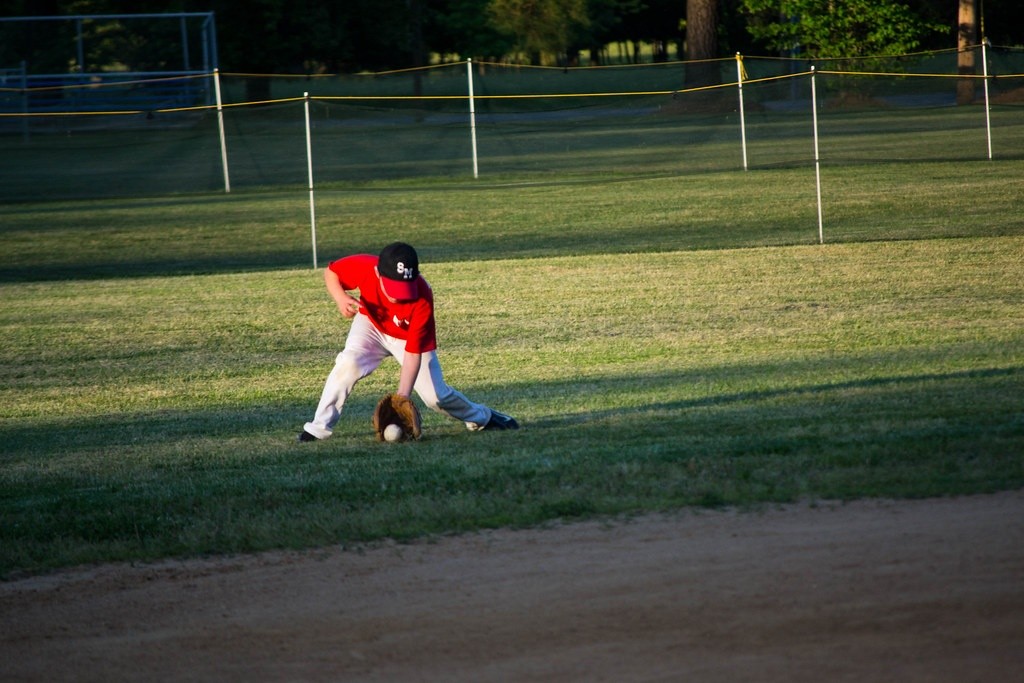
[299,242,520,444]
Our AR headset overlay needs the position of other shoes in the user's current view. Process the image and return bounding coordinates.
[483,409,519,431]
[298,430,318,443]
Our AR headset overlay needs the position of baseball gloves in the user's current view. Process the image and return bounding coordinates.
[373,391,422,443]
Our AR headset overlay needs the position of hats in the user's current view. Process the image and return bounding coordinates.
[378,242,420,300]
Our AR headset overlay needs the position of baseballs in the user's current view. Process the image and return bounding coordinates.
[383,423,403,443]
